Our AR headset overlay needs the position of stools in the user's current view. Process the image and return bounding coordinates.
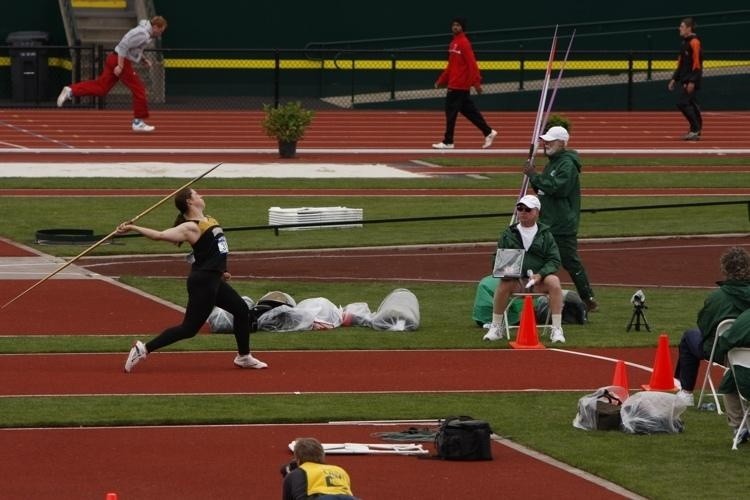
[500,289,555,343]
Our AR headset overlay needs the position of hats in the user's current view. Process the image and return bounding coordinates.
[516,195,541,212]
[540,126,569,144]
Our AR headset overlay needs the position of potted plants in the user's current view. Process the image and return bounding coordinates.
[258,97,313,159]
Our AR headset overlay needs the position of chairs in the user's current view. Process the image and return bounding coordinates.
[726,347,749,450]
[694,315,738,417]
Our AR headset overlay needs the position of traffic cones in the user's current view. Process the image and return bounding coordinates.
[508,295,547,349]
[606,357,632,402]
[638,335,680,394]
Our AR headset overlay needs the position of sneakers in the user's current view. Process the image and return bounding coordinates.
[673,378,694,406]
[132,120,154,131]
[57,86,72,108]
[550,328,565,344]
[233,353,267,370]
[482,324,503,341]
[482,130,497,149]
[683,132,700,141]
[123,340,147,373]
[583,296,595,311]
[733,428,747,445]
[431,142,453,149]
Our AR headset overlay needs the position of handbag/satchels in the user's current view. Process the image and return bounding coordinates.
[578,401,622,430]
[436,414,494,461]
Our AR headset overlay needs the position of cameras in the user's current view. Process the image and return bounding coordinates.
[280,458,297,478]
[632,290,646,305]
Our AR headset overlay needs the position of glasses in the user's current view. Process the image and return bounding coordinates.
[517,206,531,212]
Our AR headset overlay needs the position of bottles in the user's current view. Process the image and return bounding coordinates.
[701,402,715,411]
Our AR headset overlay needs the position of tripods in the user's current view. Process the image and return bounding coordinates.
[626,304,651,332]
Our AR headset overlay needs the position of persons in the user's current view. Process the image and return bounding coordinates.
[667,17,703,141]
[431,17,498,149]
[115,186,270,372]
[281,437,353,500]
[673,245,750,408]
[523,125,598,310]
[482,193,567,345]
[56,15,169,133]
[715,309,750,447]
